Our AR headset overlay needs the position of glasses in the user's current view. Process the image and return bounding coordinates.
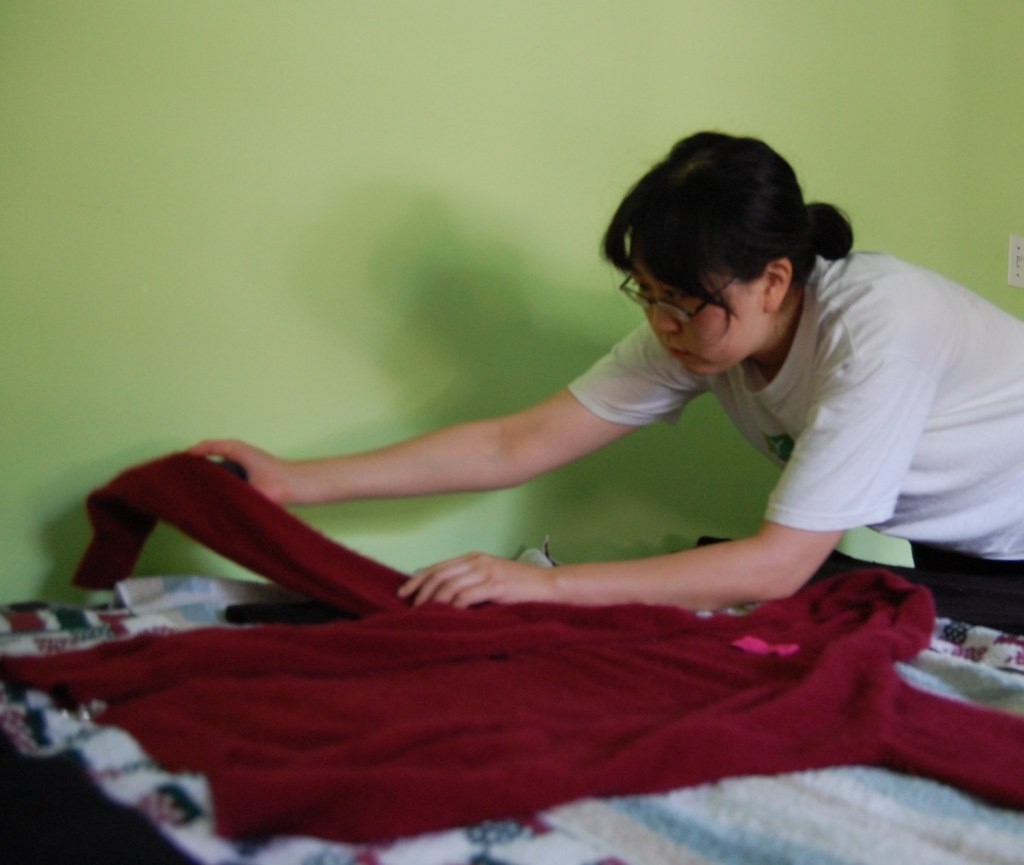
[620,273,737,322]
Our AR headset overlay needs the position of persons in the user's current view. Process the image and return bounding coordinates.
[185,131,1024,635]
